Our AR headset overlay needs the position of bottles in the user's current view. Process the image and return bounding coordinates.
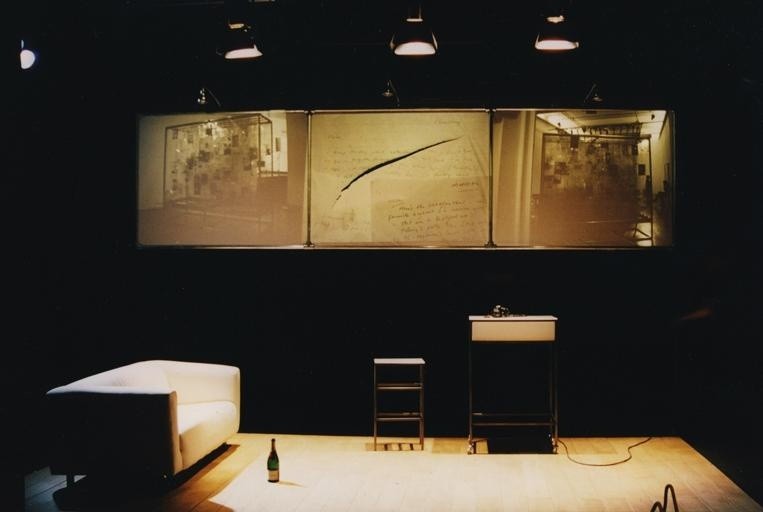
[267,438,280,483]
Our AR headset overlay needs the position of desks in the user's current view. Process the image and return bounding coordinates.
[463,313,524,452]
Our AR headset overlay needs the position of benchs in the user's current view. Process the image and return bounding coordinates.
[45,358,240,491]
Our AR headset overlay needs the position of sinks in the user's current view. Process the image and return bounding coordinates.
[468,314,558,341]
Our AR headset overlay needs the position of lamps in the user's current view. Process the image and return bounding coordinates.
[382,79,400,108]
[389,0,437,58]
[584,83,602,107]
[216,15,262,60]
[535,0,579,51]
[197,87,223,113]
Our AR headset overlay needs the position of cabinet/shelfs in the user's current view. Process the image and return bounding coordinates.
[373,356,427,451]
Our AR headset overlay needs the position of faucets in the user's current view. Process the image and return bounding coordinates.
[492,305,508,318]
